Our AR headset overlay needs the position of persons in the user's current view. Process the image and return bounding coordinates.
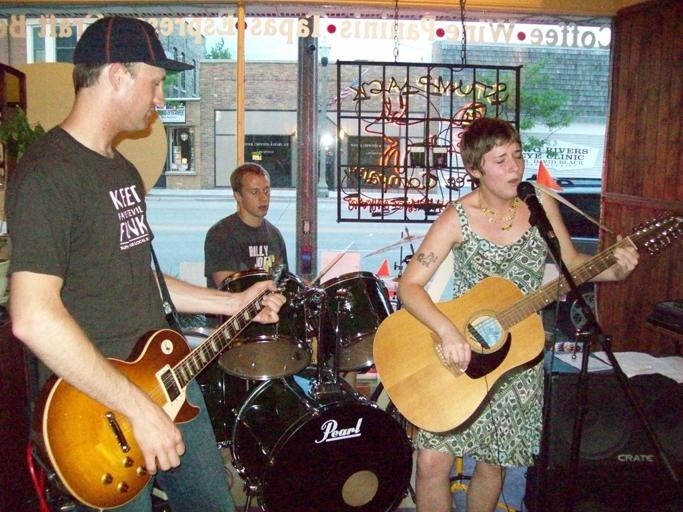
[394,116,642,512]
[200,161,317,334]
[0,14,238,512]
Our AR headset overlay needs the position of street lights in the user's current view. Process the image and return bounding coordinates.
[317,35,332,197]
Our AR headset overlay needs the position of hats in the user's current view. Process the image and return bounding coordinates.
[71,16,195,73]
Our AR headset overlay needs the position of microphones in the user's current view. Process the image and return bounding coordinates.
[517,181,560,249]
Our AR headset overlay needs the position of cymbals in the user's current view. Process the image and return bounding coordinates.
[365,233,424,259]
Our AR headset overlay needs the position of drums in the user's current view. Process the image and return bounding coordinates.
[309,272,394,373]
[181,326,265,448]
[230,365,412,511]
[216,269,311,381]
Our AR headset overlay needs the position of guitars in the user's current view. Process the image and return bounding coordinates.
[33,265,285,511]
[372,212,682,434]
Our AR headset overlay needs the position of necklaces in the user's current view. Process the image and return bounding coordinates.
[476,188,520,231]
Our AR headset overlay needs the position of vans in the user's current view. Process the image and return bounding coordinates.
[526,174,602,256]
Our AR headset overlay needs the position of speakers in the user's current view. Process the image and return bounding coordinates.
[524,350,683,512]
